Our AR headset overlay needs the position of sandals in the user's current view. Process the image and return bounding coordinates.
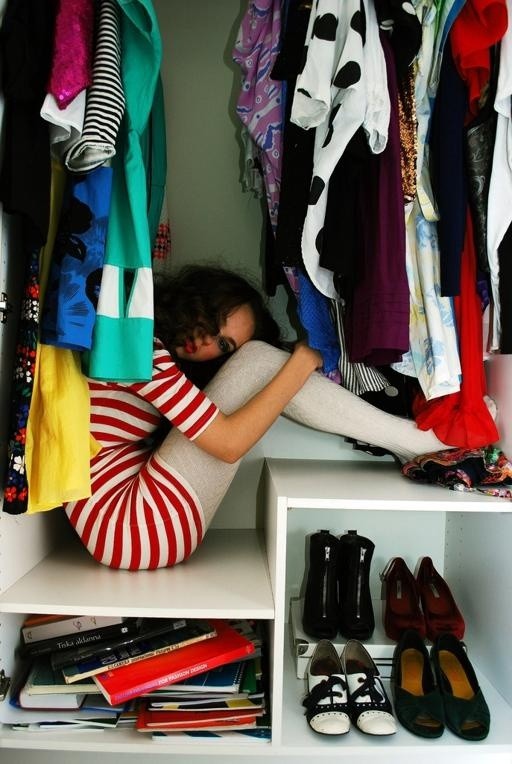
[429,631,491,742]
[389,631,445,740]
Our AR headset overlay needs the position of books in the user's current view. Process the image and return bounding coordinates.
[1,614,270,749]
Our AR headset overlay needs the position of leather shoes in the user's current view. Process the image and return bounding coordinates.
[302,638,351,737]
[383,557,427,643]
[340,638,398,736]
[416,555,466,645]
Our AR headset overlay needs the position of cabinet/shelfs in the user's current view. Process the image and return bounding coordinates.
[1,457,512,759]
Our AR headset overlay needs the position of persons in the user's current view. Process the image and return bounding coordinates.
[53,256,500,571]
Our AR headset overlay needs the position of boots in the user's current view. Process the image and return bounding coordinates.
[302,529,340,641]
[338,529,375,642]
